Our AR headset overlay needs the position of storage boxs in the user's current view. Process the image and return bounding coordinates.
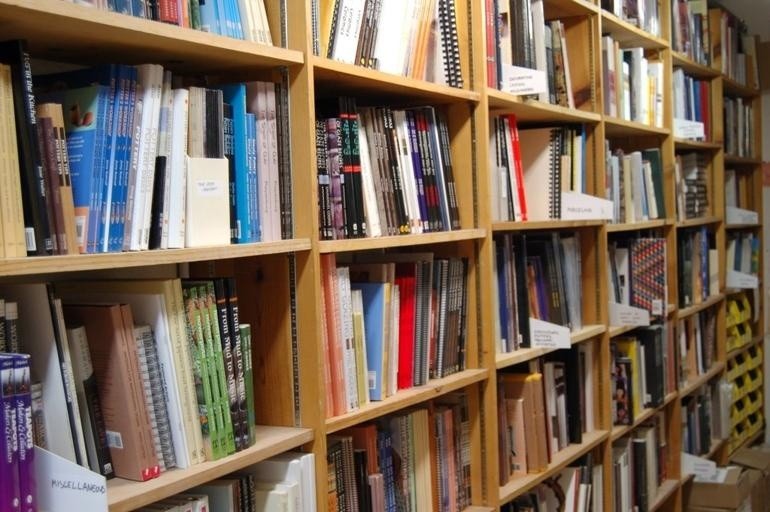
[732,448,770,505]
[684,465,751,509]
[686,468,770,512]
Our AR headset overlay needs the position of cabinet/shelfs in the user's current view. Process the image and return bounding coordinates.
[0,0,770,512]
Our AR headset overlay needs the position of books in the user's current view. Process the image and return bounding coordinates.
[726,230,761,290]
[494,340,603,487]
[728,293,753,352]
[501,452,604,511]
[311,1,465,89]
[678,227,720,308]
[675,152,710,222]
[723,94,756,159]
[2,262,255,510]
[724,346,764,457]
[489,112,587,225]
[2,47,81,260]
[611,410,669,512]
[65,1,273,46]
[326,393,474,512]
[608,326,670,425]
[602,0,664,39]
[725,169,759,224]
[674,67,713,144]
[322,252,470,418]
[680,382,715,457]
[672,0,714,67]
[602,32,665,129]
[30,64,292,254]
[722,9,760,89]
[605,138,667,225]
[315,96,459,240]
[136,452,316,511]
[485,1,577,112]
[675,304,718,391]
[606,230,667,326]
[492,229,583,354]
